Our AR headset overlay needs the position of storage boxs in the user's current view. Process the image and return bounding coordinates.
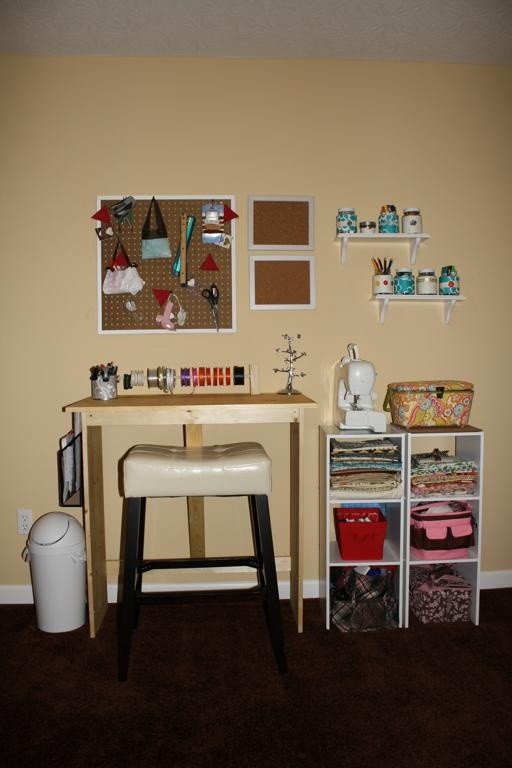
[332,507,389,562]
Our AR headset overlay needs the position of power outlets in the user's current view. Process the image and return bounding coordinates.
[15,508,35,537]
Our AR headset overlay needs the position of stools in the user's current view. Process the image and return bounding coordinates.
[112,438,289,683]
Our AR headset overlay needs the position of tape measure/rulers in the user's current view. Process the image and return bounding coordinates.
[179,213,187,285]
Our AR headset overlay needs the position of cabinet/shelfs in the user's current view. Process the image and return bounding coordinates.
[388,420,486,630]
[315,422,408,635]
[332,231,467,328]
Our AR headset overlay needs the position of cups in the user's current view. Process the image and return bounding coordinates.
[437,275,460,295]
[90,374,118,400]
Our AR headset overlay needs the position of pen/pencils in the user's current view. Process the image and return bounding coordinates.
[90,361,118,382]
[371,257,393,275]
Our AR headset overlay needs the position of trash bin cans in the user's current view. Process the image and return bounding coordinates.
[22,511,87,633]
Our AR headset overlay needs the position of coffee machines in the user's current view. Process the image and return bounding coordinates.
[332,342,387,435]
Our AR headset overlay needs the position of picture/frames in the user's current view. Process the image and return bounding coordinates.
[95,193,239,336]
[246,193,315,252]
[247,254,317,312]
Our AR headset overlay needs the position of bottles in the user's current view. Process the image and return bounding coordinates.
[371,268,394,295]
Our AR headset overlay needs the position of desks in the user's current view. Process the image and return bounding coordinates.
[60,389,319,642]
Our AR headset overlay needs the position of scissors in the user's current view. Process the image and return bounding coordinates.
[202,284,219,333]
